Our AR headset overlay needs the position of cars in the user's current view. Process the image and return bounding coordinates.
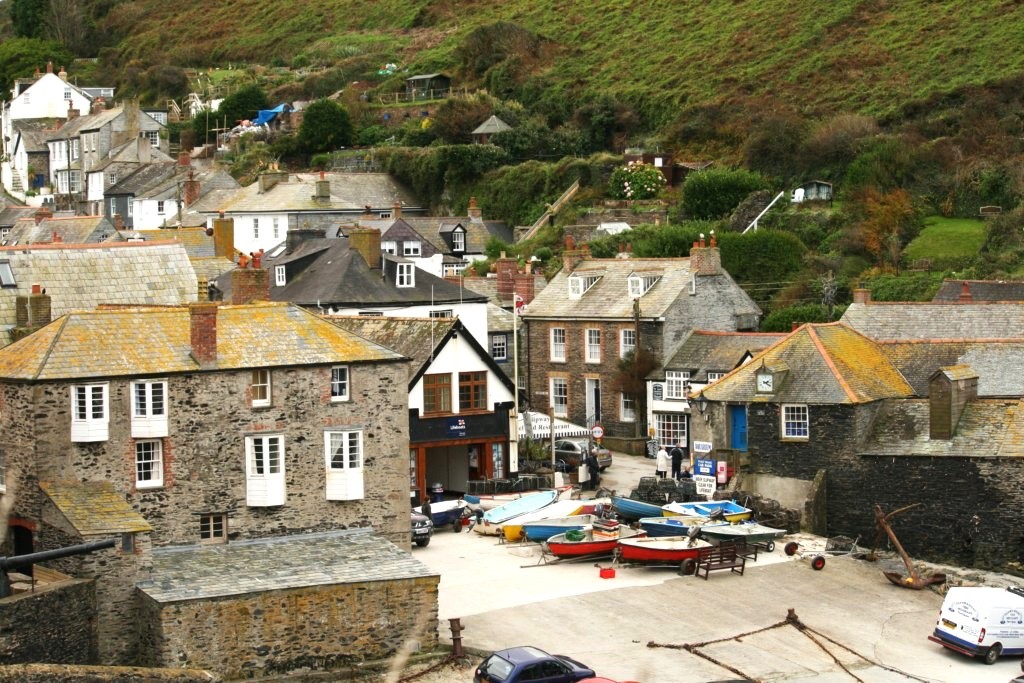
[544,438,613,471]
[473,645,596,683]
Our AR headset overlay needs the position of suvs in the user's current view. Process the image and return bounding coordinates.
[410,507,434,547]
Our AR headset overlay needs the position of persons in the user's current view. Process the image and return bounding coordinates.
[682,466,690,479]
[656,444,672,478]
[422,495,432,520]
[670,443,684,481]
[588,449,599,490]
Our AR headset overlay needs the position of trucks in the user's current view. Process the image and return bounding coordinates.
[930,586,1024,665]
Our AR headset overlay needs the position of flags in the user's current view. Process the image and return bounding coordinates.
[515,295,527,315]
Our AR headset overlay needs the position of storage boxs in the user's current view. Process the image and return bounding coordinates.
[592,529,619,542]
[566,528,586,542]
[599,568,615,579]
[592,519,620,534]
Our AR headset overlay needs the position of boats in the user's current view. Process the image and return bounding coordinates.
[520,514,601,541]
[616,534,714,563]
[546,522,648,557]
[501,496,586,539]
[413,498,467,527]
[662,499,753,524]
[637,514,741,538]
[611,494,664,525]
[696,523,789,545]
[467,488,560,536]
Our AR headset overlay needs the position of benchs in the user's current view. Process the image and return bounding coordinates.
[694,546,746,581]
[719,535,757,564]
